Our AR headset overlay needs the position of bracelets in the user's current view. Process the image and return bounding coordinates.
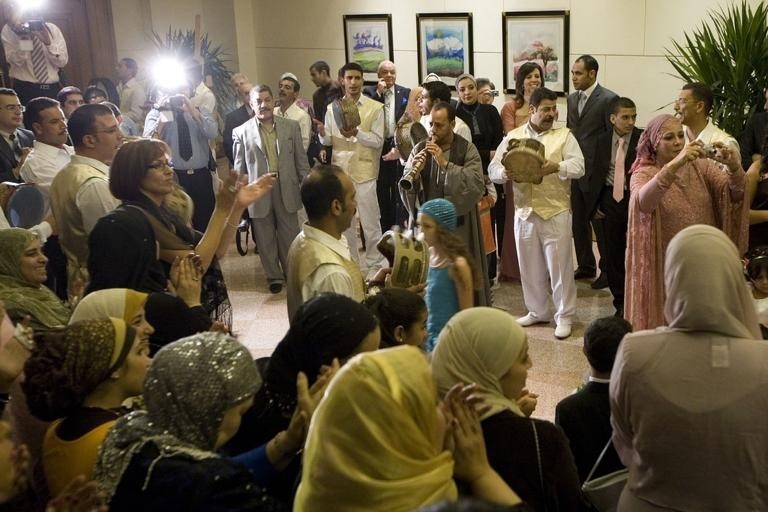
[727,167,739,173]
[226,216,245,227]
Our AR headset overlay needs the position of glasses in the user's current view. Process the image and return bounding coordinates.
[2,105,25,114]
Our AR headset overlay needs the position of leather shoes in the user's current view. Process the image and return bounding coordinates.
[554,324,571,339]
[516,311,550,327]
[269,283,282,294]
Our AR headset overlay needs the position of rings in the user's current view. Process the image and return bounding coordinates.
[192,278,199,280]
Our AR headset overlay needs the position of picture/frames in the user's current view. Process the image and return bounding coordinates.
[342,14,395,74]
[501,10,570,99]
[415,12,474,91]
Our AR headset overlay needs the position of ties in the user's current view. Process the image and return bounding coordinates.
[8,135,23,163]
[612,137,626,203]
[174,111,197,160]
[32,31,48,83]
[578,92,587,115]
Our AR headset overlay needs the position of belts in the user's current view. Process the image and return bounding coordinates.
[18,79,60,89]
[176,166,208,175]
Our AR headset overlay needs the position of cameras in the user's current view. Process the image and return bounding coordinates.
[167,96,183,107]
[489,90,499,96]
[699,144,717,159]
[21,20,42,33]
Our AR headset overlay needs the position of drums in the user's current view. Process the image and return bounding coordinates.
[395,120,429,160]
[502,139,546,183]
[333,97,361,134]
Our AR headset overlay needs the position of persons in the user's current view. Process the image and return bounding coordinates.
[414,198,477,353]
[273,55,767,338]
[1,55,311,510]
[608,226,766,510]
[0,6,69,108]
[285,164,428,324]
[249,287,633,508]
